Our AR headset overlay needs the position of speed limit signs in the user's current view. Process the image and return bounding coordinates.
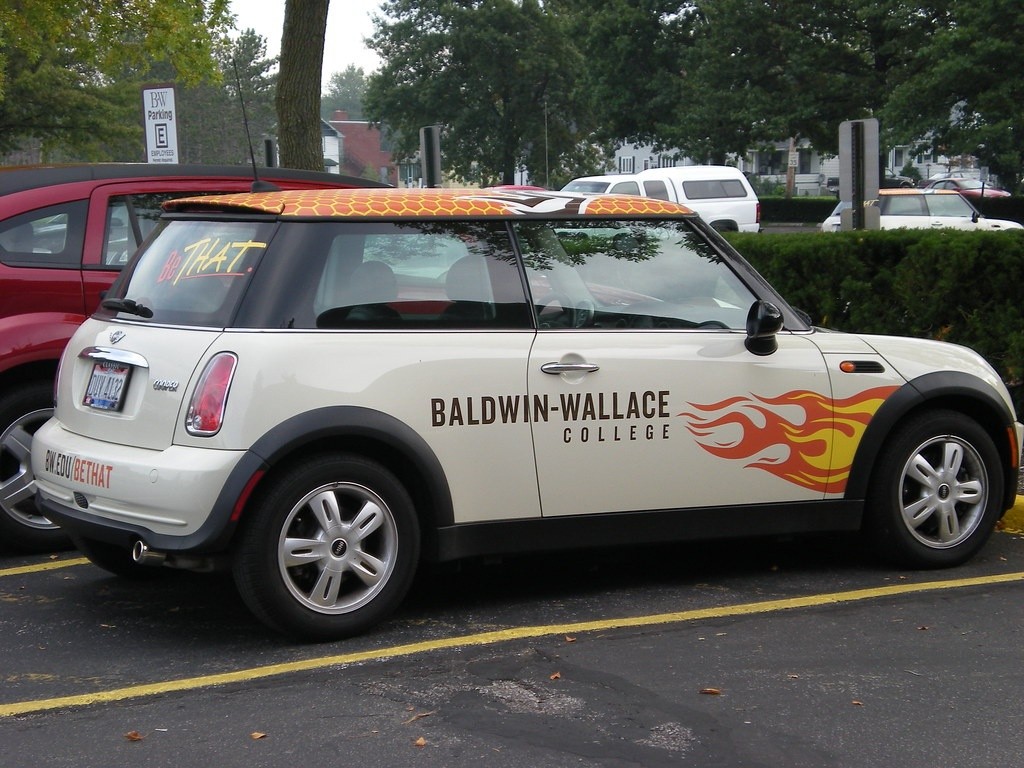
[788,152,800,168]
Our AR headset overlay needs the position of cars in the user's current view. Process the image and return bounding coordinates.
[923,177,1013,198]
[26,182,1024,647]
[919,171,971,187]
[816,187,1024,235]
[28,204,164,265]
[1,161,662,554]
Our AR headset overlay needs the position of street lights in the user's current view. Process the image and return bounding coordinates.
[925,164,932,179]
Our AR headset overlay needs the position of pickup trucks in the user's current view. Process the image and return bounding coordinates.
[827,167,915,199]
[558,166,766,235]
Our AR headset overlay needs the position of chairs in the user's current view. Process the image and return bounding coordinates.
[347,260,404,328]
[436,254,496,328]
